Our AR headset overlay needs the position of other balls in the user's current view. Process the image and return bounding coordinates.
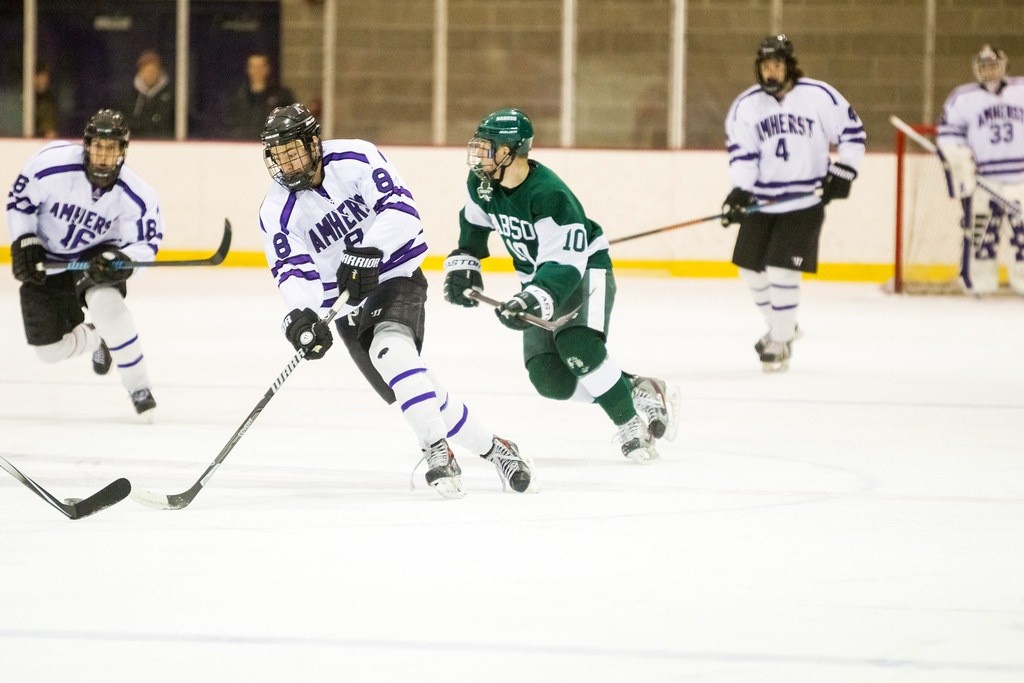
[64,498,83,505]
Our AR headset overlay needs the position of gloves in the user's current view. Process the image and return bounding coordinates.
[495,285,557,330]
[335,246,383,306]
[85,251,133,286]
[821,162,857,203]
[721,188,756,227]
[282,307,334,360]
[10,234,49,287]
[443,249,483,307]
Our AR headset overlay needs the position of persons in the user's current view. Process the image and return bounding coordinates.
[936,44,1024,297]
[721,34,865,374]
[0,50,296,140]
[260,103,538,499]
[444,108,678,464]
[8,109,164,423]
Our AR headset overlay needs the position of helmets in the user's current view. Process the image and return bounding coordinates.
[754,35,793,94]
[262,103,324,192]
[467,109,533,182]
[972,45,1009,93]
[82,109,130,187]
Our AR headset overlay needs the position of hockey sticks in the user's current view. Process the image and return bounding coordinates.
[888,112,1023,219]
[464,288,597,332]
[0,455,132,520]
[35,217,234,272]
[607,187,823,245]
[128,288,352,512]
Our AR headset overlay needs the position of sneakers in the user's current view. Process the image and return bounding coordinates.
[755,322,799,373]
[422,439,466,499]
[618,373,679,464]
[479,435,540,493]
[129,386,156,424]
[87,323,112,374]
